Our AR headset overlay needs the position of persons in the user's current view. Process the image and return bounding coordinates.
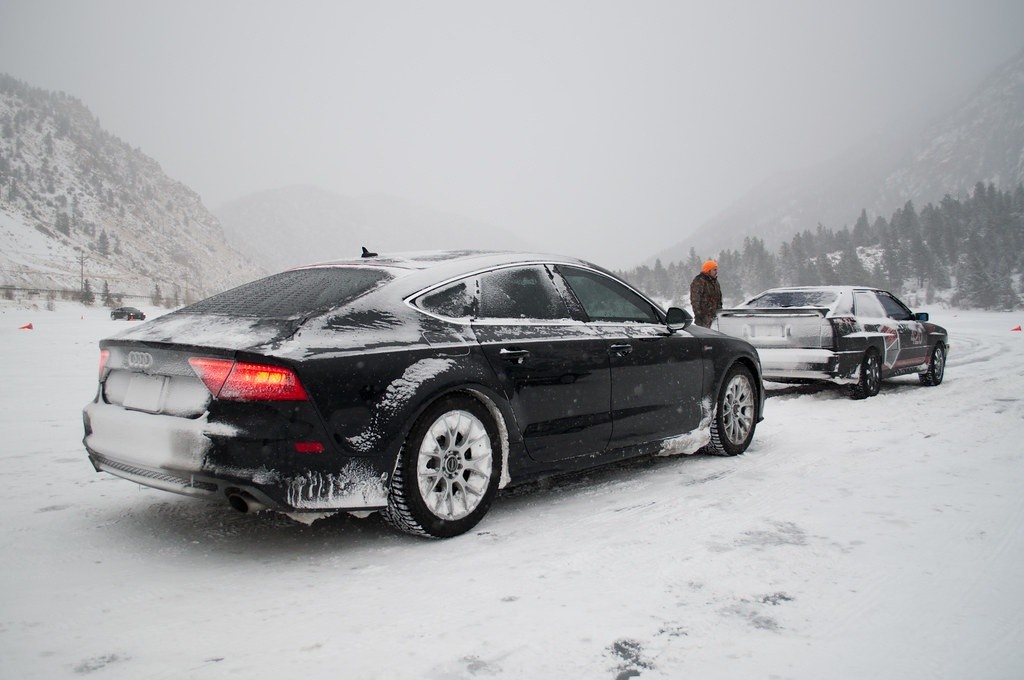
[690,261,723,328]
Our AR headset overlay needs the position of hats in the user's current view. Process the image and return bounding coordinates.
[702,261,718,274]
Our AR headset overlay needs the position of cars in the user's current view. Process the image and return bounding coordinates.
[709,284,951,401]
[82,246,768,541]
[109,306,147,322]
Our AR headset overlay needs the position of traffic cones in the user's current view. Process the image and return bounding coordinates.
[19,323,35,329]
[1010,325,1022,330]
